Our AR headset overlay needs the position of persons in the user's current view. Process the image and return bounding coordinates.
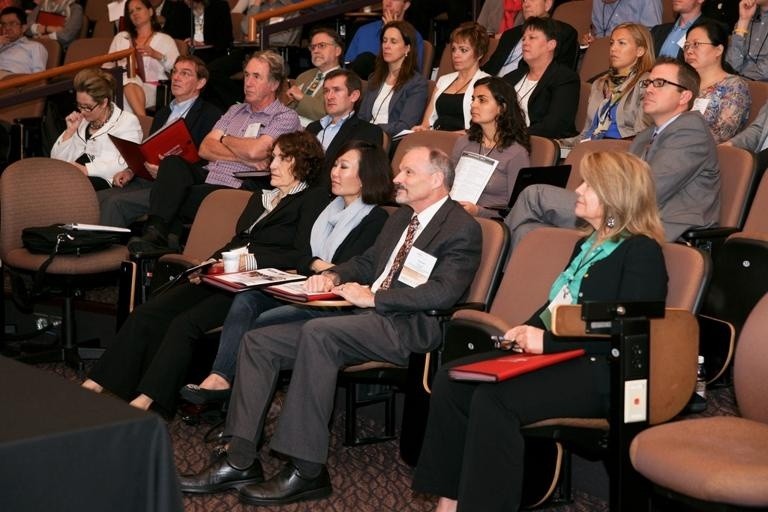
[1,0,768,510]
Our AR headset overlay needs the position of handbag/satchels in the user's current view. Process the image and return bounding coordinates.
[22,224,112,255]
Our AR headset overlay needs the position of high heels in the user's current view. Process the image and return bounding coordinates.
[180,384,233,412]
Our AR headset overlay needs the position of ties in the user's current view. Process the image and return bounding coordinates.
[307,73,323,95]
[381,216,420,290]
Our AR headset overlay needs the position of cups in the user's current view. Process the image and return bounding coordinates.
[222,252,240,273]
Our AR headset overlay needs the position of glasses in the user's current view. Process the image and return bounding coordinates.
[76,104,97,112]
[685,43,713,48]
[639,79,686,90]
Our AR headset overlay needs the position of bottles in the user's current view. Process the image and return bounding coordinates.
[696,356,706,399]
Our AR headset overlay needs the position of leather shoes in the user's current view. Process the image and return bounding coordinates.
[128,233,159,253]
[240,461,333,506]
[178,451,263,493]
